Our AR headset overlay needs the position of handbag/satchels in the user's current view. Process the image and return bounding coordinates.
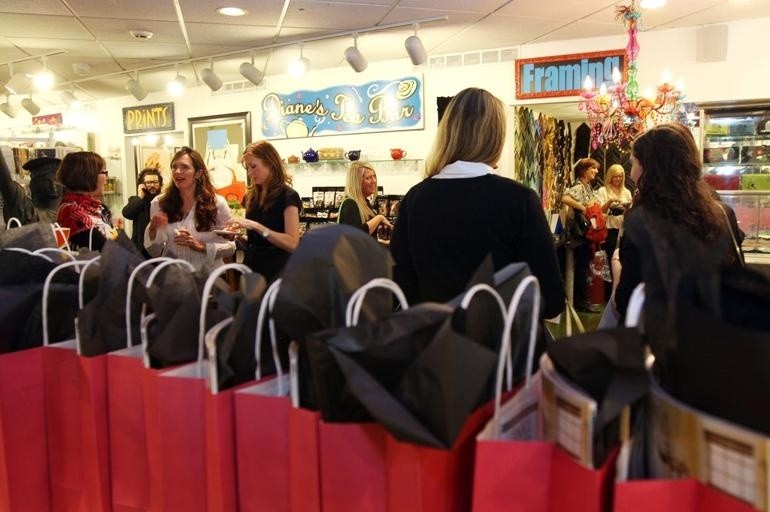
[470,276,646,512]
[320,278,413,512]
[388,269,510,511]
[104,258,196,510]
[38,256,107,511]
[710,197,770,334]
[0,347,51,512]
[234,278,318,512]
[144,258,251,512]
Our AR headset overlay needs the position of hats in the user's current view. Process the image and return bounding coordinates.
[22,157,62,179]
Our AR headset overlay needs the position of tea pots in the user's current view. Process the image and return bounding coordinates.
[280,116,318,139]
[301,148,320,162]
[389,148,407,160]
[345,149,362,160]
[288,155,300,164]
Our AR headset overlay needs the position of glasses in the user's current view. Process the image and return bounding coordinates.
[97,170,109,176]
[142,180,161,186]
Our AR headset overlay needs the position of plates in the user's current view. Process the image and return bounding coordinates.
[213,229,242,237]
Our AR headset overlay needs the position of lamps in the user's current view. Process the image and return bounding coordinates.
[7,65,31,96]
[2,93,17,120]
[579,0,698,156]
[240,53,265,88]
[126,72,148,104]
[202,59,224,93]
[22,90,43,117]
[405,24,429,69]
[343,33,371,75]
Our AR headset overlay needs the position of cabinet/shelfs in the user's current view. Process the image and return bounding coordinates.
[692,107,770,253]
[297,186,404,238]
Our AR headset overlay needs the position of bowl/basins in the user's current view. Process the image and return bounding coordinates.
[320,149,344,160]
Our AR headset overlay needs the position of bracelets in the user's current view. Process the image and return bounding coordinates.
[612,257,621,262]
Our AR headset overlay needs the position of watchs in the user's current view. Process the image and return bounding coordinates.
[262,229,271,241]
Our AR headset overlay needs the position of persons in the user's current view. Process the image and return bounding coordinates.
[122,167,162,254]
[337,161,393,246]
[610,122,745,375]
[217,141,301,271]
[57,151,113,251]
[1,152,61,227]
[555,157,600,314]
[143,147,237,275]
[390,88,566,354]
[596,164,632,298]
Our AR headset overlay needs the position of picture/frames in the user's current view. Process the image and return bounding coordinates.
[188,111,250,212]
